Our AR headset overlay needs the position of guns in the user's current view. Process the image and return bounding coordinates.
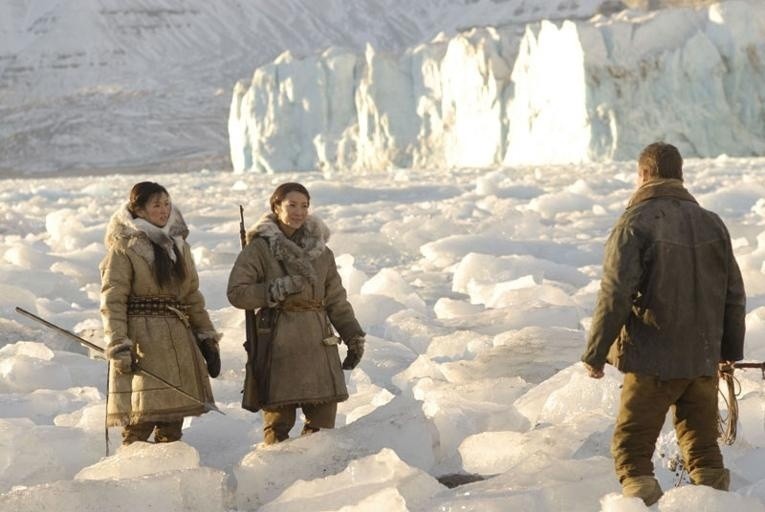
[239,206,261,413]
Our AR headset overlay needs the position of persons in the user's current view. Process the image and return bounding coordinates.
[579,143,748,507]
[98,179,224,448]
[226,181,365,446]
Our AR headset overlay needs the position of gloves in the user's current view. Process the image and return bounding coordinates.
[270,275,304,303]
[200,339,220,378]
[112,349,136,373]
[342,336,364,369]
